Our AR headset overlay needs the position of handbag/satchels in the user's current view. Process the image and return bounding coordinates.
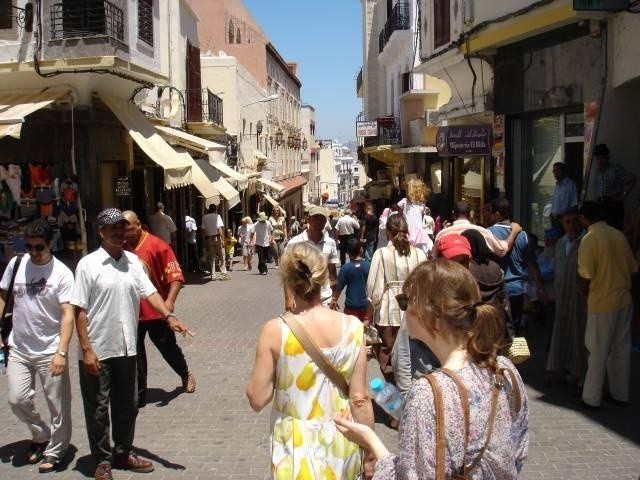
[0,254,23,367]
[365,326,382,345]
[361,367,501,480]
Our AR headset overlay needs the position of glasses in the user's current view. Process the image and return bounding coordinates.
[23,242,46,251]
[395,293,411,310]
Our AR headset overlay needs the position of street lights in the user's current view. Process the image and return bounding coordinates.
[239,94,280,139]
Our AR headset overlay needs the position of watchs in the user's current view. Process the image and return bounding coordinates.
[165,313,177,319]
[56,349,68,357]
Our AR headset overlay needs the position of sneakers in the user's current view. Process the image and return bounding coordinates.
[182,373,195,392]
[139,389,150,406]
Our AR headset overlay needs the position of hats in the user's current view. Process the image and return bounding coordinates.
[256,212,268,221]
[97,207,130,227]
[435,235,471,259]
[308,206,327,219]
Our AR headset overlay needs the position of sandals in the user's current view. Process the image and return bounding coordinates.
[26,439,43,461]
[39,456,62,472]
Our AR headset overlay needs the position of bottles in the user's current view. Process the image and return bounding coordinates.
[0,348,6,375]
[369,377,406,421]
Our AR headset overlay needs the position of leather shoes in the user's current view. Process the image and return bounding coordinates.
[95,464,113,480]
[120,455,153,473]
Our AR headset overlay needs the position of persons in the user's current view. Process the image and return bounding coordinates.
[250,212,273,275]
[460,229,513,349]
[0,219,74,472]
[223,229,236,271]
[378,204,398,248]
[333,258,529,480]
[237,217,254,271]
[423,207,435,239]
[282,205,339,313]
[479,200,492,228]
[246,216,256,256]
[329,214,338,238]
[288,215,299,238]
[360,205,377,260]
[335,210,359,263]
[527,227,562,303]
[322,219,332,239]
[246,242,376,480]
[546,208,591,373]
[486,198,549,335]
[147,202,177,249]
[435,201,522,258]
[398,179,430,254]
[200,204,228,281]
[575,202,639,409]
[366,215,427,433]
[70,208,194,480]
[269,206,287,268]
[549,163,578,237]
[329,237,371,322]
[389,234,472,398]
[120,211,196,409]
[588,143,636,229]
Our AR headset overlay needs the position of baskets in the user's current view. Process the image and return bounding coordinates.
[506,336,530,365]
